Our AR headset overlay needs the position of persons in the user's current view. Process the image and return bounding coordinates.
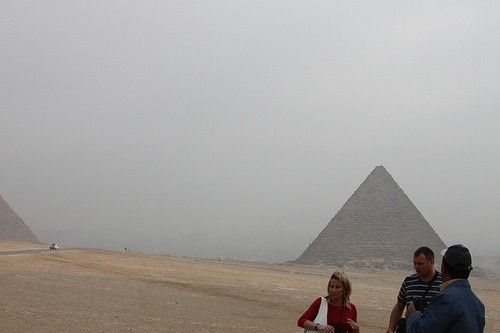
[405,244,485,333]
[386,246,444,333]
[297,271,359,333]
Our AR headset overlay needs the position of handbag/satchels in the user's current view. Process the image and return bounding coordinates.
[395,317,407,333]
[305,297,327,333]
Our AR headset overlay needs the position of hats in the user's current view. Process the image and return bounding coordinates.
[441,244,473,270]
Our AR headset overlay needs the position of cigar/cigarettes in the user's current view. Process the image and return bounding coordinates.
[406,300,413,304]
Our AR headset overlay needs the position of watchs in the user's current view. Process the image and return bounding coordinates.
[315,323,319,331]
